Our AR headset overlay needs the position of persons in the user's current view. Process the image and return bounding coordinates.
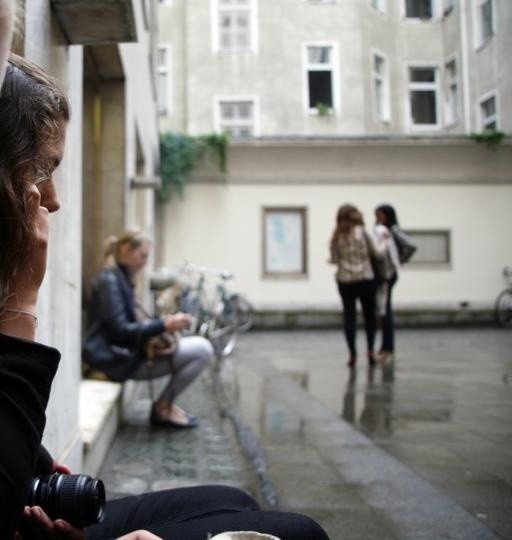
[330,204,384,366]
[83,224,213,428]
[115,529,163,540]
[1,45,330,540]
[375,205,417,365]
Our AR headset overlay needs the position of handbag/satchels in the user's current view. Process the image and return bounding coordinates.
[361,226,385,289]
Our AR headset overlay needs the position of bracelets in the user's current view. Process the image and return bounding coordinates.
[5,308,37,319]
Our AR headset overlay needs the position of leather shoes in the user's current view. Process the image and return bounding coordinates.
[150,402,199,429]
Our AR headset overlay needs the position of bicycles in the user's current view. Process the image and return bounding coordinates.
[177,269,257,334]
[493,266,512,327]
[166,256,241,360]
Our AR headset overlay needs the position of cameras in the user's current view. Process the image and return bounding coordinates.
[25,469,106,529]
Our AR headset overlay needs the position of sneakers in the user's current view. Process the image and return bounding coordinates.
[368,350,395,368]
[348,354,357,367]
